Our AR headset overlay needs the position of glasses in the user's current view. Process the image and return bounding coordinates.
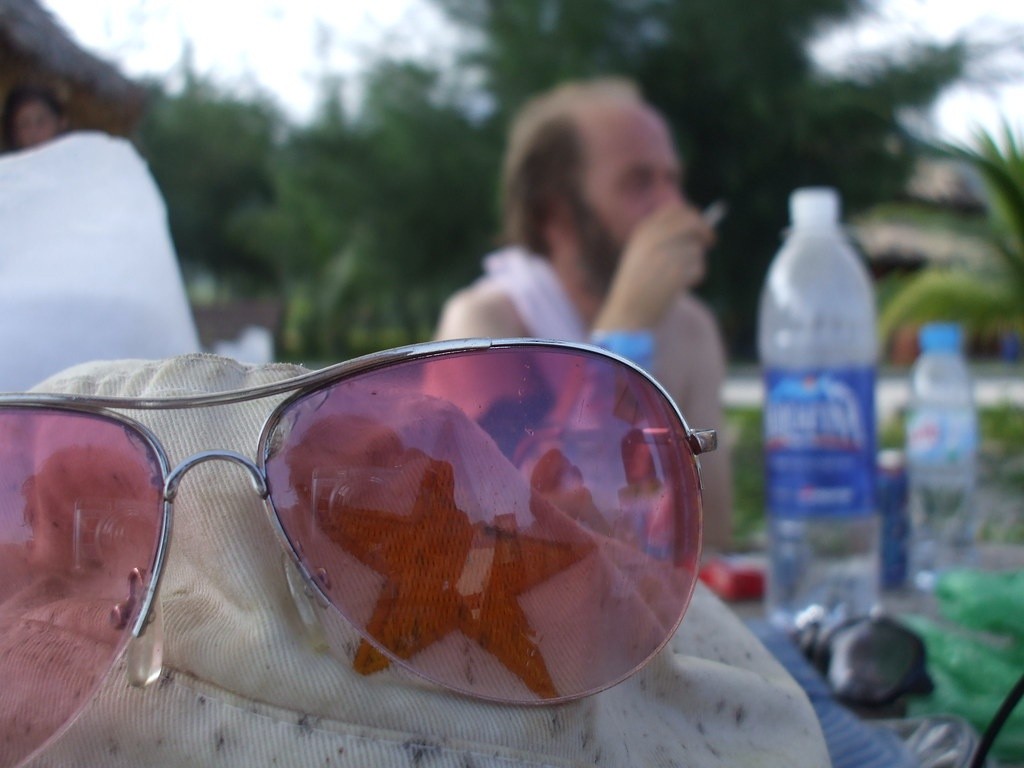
[0,335,715,768]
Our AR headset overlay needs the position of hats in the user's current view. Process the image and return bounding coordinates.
[0,353,827,768]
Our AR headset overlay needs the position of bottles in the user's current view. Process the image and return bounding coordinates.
[758,184,879,646]
[908,326,977,592]
[880,448,908,596]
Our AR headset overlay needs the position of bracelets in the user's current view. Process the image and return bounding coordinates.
[588,329,656,384]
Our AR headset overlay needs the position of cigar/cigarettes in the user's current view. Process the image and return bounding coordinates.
[701,196,725,229]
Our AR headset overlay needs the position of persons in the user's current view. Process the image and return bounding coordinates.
[418,76,733,563]
[529,428,672,562]
[4,85,69,152]
[0,442,149,630]
